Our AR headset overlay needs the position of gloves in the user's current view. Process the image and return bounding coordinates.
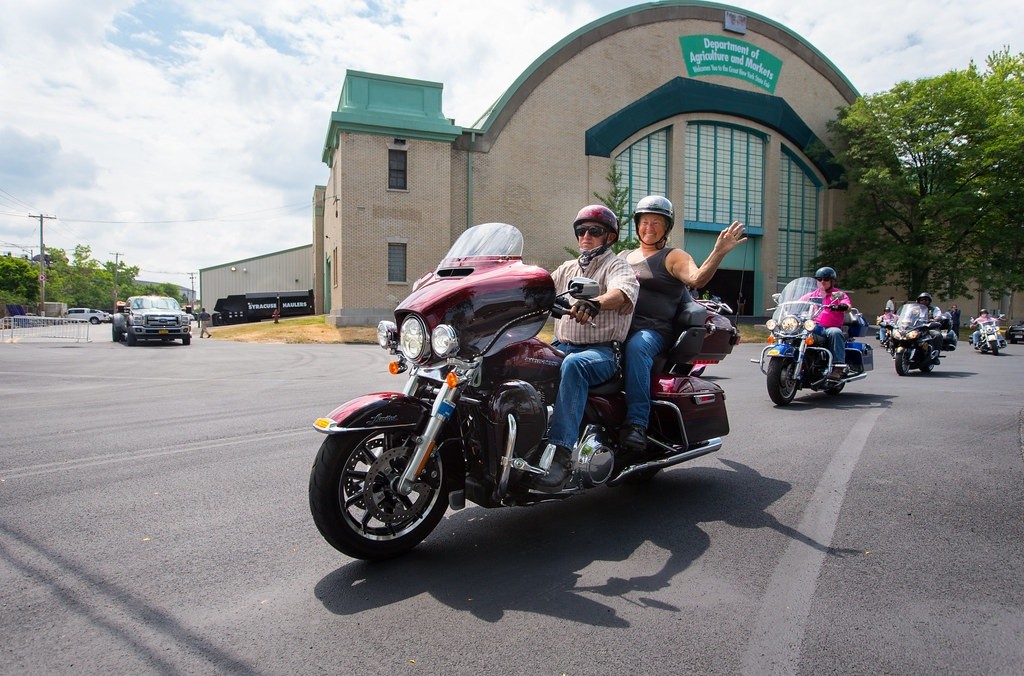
[837,303,849,311]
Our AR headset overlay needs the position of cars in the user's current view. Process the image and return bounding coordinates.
[0,313,37,330]
[188,313,199,321]
[90,310,113,323]
[1006,320,1024,344]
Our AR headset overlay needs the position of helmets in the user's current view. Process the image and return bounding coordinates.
[884,307,890,312]
[570,204,619,234]
[980,309,988,315]
[815,267,837,290]
[633,194,675,222]
[917,293,933,302]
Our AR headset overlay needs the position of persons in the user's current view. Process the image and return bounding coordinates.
[950,303,961,340]
[535,205,639,493]
[796,266,852,380]
[886,296,896,313]
[879,306,900,343]
[970,308,1007,348]
[614,195,747,448]
[198,308,212,338]
[916,292,941,365]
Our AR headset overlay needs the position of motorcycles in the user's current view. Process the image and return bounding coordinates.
[879,300,958,376]
[970,313,1008,355]
[309,221,741,560]
[751,277,874,404]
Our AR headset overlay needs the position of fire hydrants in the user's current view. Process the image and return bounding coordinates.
[272,310,282,323]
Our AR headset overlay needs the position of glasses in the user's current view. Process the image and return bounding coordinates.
[575,225,608,237]
[818,277,828,282]
[920,297,929,301]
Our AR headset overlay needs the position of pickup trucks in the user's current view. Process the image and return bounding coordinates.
[65,308,109,324]
[114,296,192,345]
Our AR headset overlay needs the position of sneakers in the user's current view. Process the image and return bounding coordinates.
[828,368,847,381]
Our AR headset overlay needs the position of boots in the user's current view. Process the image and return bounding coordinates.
[531,445,575,494]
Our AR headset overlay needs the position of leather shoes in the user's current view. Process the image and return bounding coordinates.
[623,424,648,450]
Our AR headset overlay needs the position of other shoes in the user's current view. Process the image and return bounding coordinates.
[881,340,885,344]
[208,335,211,338]
